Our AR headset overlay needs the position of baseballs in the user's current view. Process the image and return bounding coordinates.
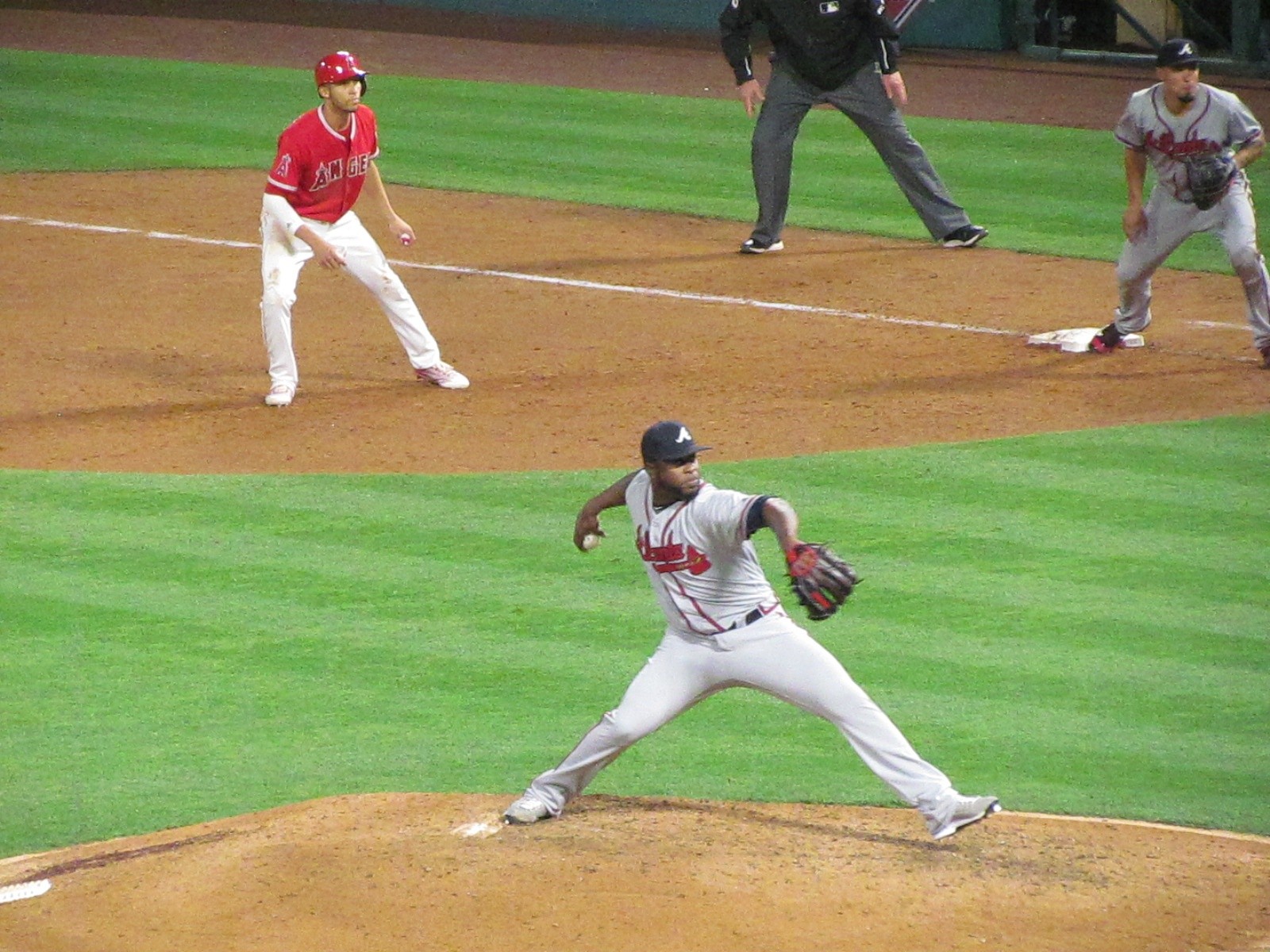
[580,534,602,548]
[400,233,410,245]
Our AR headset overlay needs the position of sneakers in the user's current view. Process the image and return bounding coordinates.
[412,361,470,392]
[939,224,990,249]
[738,235,786,254]
[264,385,297,406]
[1260,347,1270,364]
[1089,321,1129,357]
[502,795,552,827]
[928,793,1005,840]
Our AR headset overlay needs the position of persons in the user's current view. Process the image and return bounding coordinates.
[1089,37,1270,368]
[259,50,470,405]
[717,0,990,254]
[500,422,997,840]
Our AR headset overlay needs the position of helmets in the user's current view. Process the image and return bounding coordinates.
[314,49,371,89]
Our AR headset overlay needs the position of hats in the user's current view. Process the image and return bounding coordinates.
[641,420,714,463]
[1155,38,1204,68]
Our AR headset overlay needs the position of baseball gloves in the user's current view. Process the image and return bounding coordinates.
[785,544,859,620]
[1186,154,1235,211]
